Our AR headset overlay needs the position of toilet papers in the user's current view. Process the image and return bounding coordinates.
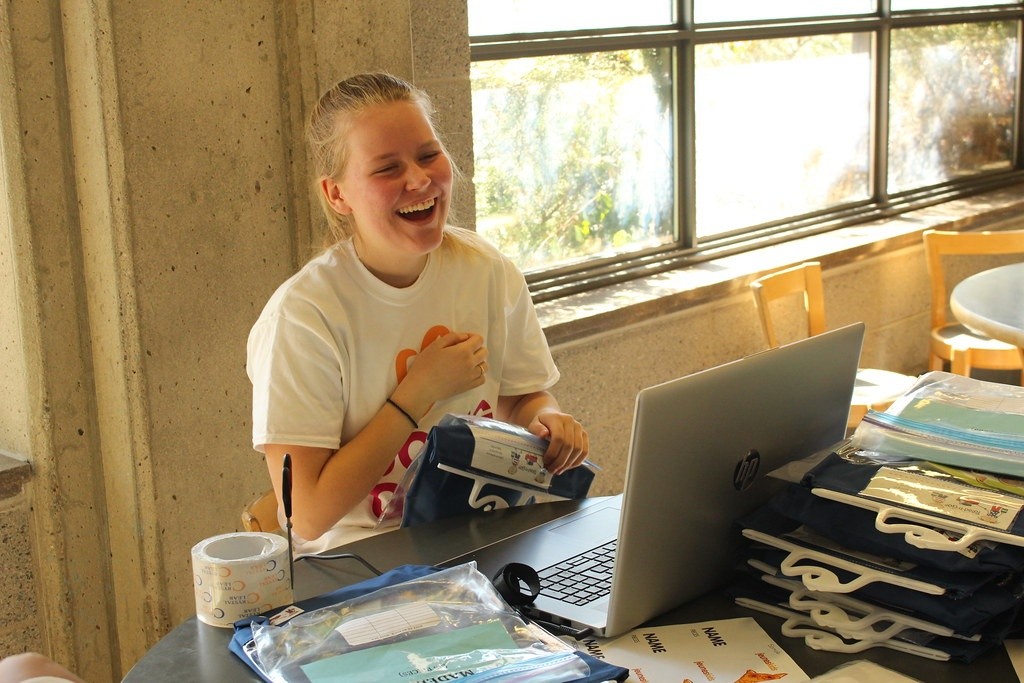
[190,530,293,628]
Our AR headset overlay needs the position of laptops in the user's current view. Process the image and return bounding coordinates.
[433,321,867,638]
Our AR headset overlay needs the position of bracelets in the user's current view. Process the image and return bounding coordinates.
[386,398,418,429]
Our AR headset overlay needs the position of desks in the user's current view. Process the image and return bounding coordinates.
[950,262,1024,348]
[120,496,1023,683]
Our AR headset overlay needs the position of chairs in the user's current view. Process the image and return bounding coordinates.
[751,261,917,428]
[922,229,1024,387]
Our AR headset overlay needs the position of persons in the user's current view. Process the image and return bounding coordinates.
[246,73,590,555]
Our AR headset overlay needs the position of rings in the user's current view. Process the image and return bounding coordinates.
[479,364,485,375]
